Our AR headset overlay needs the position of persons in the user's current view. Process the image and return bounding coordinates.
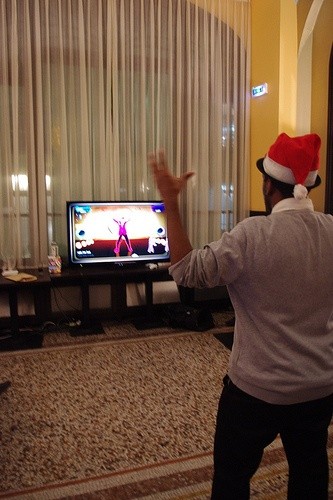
[145,132,333,500]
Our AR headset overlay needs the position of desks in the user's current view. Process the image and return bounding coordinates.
[0,263,196,336]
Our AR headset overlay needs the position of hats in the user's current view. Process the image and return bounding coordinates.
[255,131,322,201]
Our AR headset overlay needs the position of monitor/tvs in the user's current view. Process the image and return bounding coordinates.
[66,201,171,269]
